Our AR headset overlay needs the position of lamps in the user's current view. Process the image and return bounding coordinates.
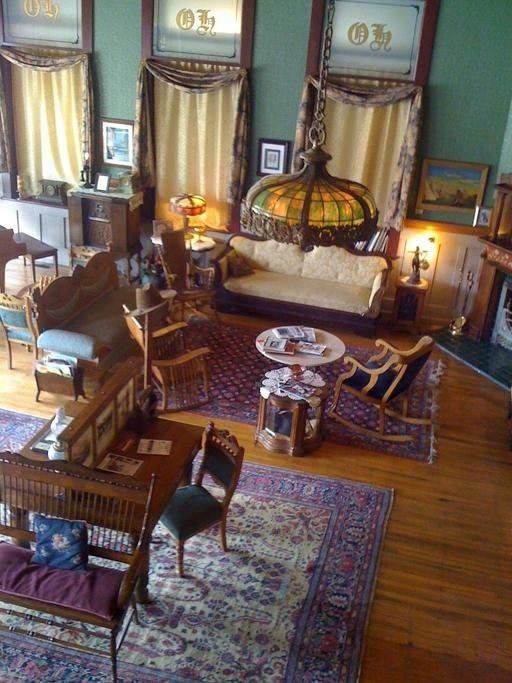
[237,1,381,256]
[169,192,207,240]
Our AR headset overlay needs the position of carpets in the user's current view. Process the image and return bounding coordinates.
[0,408,394,682]
[137,312,450,466]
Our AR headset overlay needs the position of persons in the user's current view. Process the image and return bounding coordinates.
[282,379,308,394]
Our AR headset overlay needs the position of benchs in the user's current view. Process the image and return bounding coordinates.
[0,451,157,683]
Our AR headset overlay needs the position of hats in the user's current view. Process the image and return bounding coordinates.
[125,282,178,317]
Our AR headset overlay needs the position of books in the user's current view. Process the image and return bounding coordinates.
[136,437,174,456]
[278,378,317,399]
[260,325,327,357]
[95,452,144,477]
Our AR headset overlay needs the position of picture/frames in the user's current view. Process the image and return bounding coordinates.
[255,137,290,178]
[471,204,494,228]
[93,172,112,193]
[415,157,492,215]
[97,116,135,171]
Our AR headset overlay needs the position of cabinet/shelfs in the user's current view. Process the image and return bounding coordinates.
[66,183,144,285]
